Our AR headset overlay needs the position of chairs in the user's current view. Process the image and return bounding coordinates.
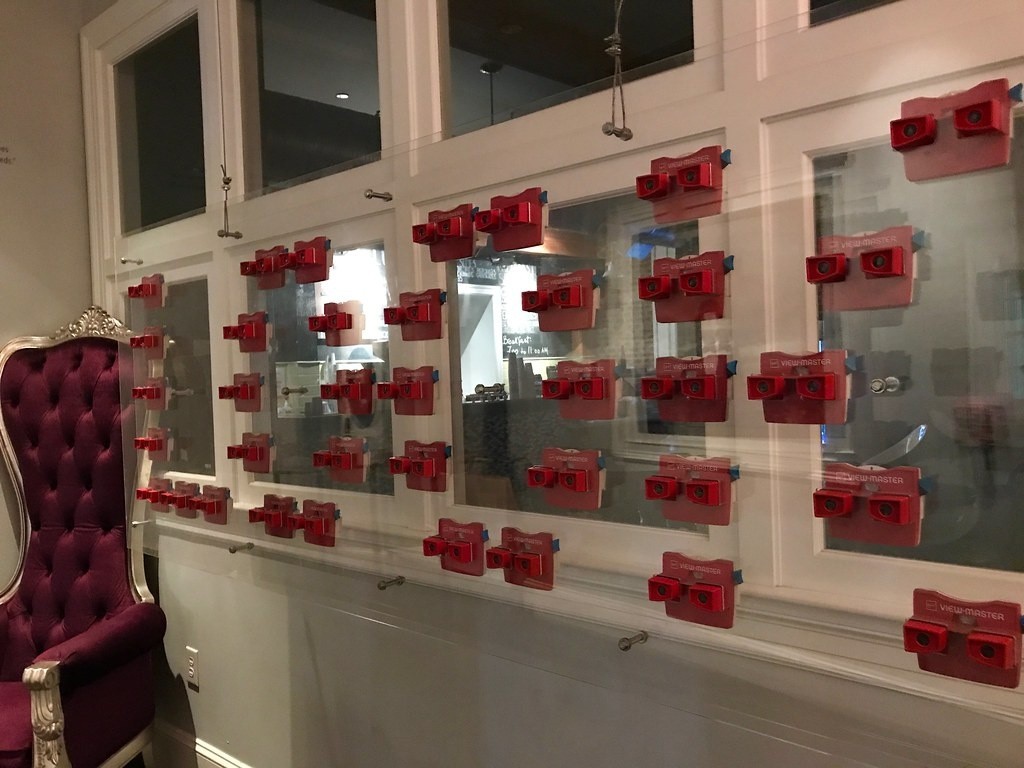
[0,306,167,768]
